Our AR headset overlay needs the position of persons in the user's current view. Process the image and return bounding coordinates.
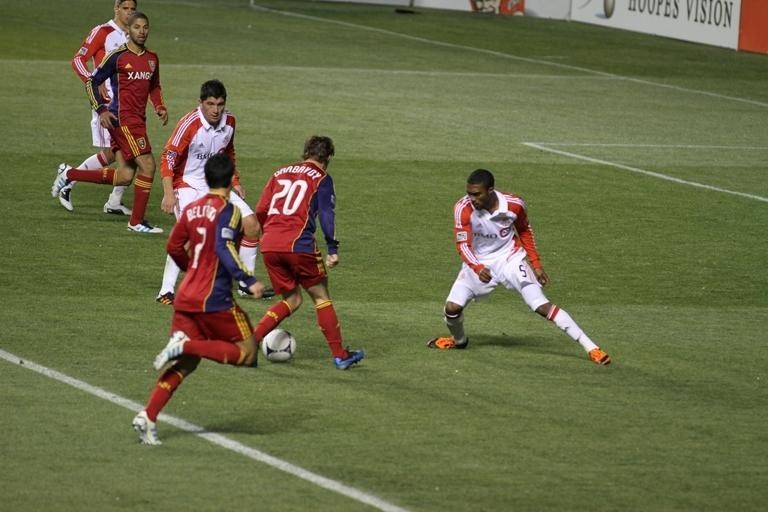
[131,155,257,446]
[51,12,168,234]
[155,80,266,305]
[426,170,611,367]
[255,135,364,370]
[58,0,137,217]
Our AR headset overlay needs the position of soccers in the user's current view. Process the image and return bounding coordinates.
[260,328,298,363]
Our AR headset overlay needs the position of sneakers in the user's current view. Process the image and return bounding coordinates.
[586,344,614,366]
[125,220,164,235]
[132,409,163,446]
[59,180,76,212]
[332,349,365,370]
[155,292,176,304]
[152,329,192,371]
[50,163,73,199]
[236,283,276,300]
[102,202,133,216]
[426,335,471,351]
